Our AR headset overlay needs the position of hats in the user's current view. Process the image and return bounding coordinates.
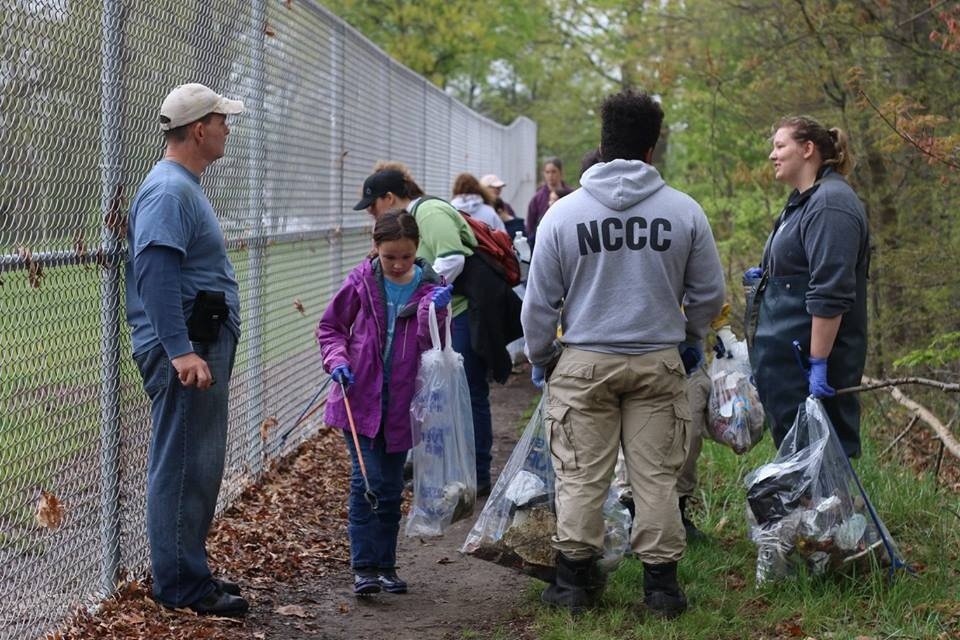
[159,82,243,131]
[479,174,506,189]
[354,169,403,210]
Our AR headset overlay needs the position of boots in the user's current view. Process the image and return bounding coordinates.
[474,453,494,498]
[618,496,636,536]
[641,561,687,619]
[676,494,706,540]
[540,549,595,611]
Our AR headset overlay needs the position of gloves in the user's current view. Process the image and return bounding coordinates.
[431,284,454,313]
[331,365,354,385]
[743,268,760,287]
[808,358,834,400]
[713,324,737,360]
[676,341,704,378]
[531,363,545,388]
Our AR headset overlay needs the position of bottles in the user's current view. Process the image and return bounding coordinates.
[548,189,558,207]
[513,230,531,262]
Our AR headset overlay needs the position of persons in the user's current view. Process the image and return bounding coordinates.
[316,153,706,593]
[742,116,872,581]
[127,83,250,614]
[518,91,726,617]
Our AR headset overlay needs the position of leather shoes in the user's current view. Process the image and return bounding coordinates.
[375,566,407,593]
[209,580,241,595]
[191,592,250,618]
[353,573,380,595]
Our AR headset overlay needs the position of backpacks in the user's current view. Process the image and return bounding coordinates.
[411,197,521,288]
